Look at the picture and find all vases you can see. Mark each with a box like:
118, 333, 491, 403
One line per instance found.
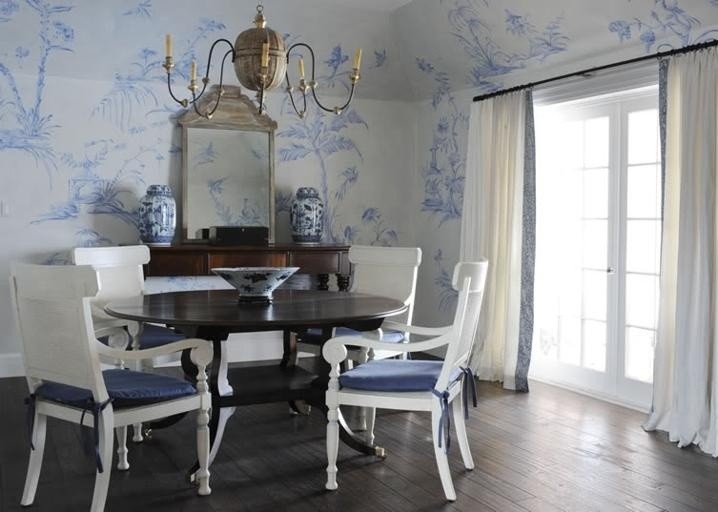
288, 186, 324, 246
136, 183, 178, 247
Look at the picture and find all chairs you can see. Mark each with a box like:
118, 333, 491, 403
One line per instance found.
295, 241, 426, 440
74, 245, 200, 442
12, 260, 214, 505
323, 256, 490, 497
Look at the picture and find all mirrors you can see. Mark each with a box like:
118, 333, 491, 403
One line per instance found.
175, 83, 278, 245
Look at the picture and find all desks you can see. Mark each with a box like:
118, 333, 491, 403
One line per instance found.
101, 286, 403, 479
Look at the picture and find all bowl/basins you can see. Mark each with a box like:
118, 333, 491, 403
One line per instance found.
211, 266, 300, 302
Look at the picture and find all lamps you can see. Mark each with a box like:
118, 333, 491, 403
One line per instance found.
161, 1, 362, 121
